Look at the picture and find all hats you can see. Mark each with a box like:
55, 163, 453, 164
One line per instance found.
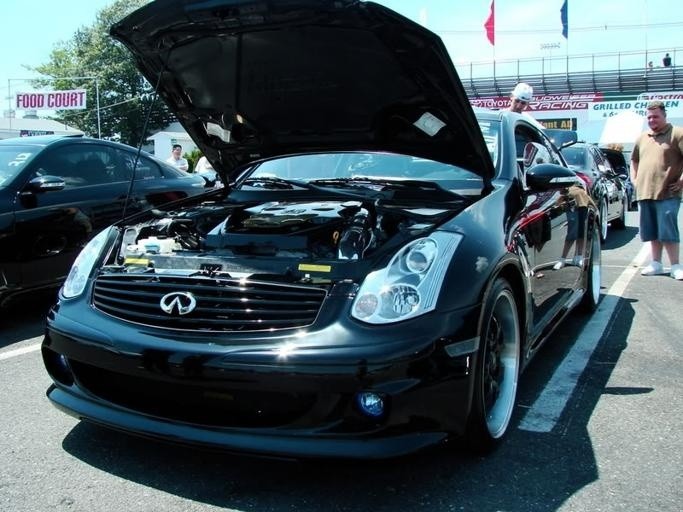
512, 82, 533, 102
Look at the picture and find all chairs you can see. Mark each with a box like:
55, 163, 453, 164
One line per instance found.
75, 158, 111, 183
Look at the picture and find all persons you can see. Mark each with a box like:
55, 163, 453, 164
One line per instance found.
662, 53, 672, 67
502, 83, 534, 113
64, 207, 93, 239
552, 186, 598, 270
630, 101, 683, 280
648, 61, 654, 71
166, 144, 218, 187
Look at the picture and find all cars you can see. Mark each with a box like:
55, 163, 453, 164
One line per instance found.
0, 134, 216, 317
600, 148, 638, 211
194, 156, 219, 184
560, 143, 629, 243
41, 0, 603, 464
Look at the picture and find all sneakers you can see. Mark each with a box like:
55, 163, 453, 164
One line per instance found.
669, 262, 683, 280
641, 259, 664, 276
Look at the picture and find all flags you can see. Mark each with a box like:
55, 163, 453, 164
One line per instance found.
484, 0, 495, 46
560, 0, 568, 40
